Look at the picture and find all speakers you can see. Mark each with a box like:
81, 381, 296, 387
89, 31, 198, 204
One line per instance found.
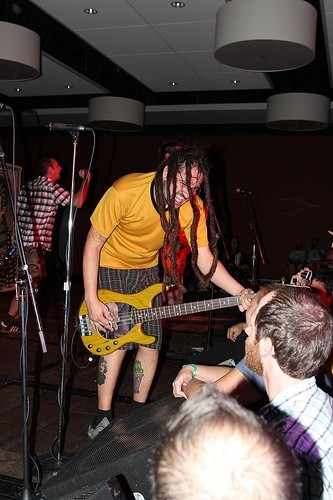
32, 391, 189, 500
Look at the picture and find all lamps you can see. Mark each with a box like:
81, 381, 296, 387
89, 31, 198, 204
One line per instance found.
265, 93, 331, 133
86, 95, 146, 136
0, 20, 43, 84
213, 0, 319, 73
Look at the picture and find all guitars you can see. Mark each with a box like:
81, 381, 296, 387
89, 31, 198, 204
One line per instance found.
76, 278, 312, 357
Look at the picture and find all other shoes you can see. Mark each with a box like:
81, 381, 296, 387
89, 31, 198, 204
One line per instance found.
4, 314, 22, 326
87, 415, 110, 438
0, 321, 21, 334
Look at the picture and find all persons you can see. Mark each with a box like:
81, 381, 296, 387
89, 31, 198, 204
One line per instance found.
307, 236, 325, 263
161, 204, 209, 305
289, 240, 307, 269
223, 238, 251, 273
80, 137, 255, 439
0, 156, 91, 332
244, 285, 333, 500
146, 381, 304, 500
172, 267, 333, 421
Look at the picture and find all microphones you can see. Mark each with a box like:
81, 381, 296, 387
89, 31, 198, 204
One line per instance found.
236, 188, 254, 195
44, 121, 92, 132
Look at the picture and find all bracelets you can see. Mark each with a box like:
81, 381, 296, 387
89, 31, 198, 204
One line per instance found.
182, 363, 199, 379
